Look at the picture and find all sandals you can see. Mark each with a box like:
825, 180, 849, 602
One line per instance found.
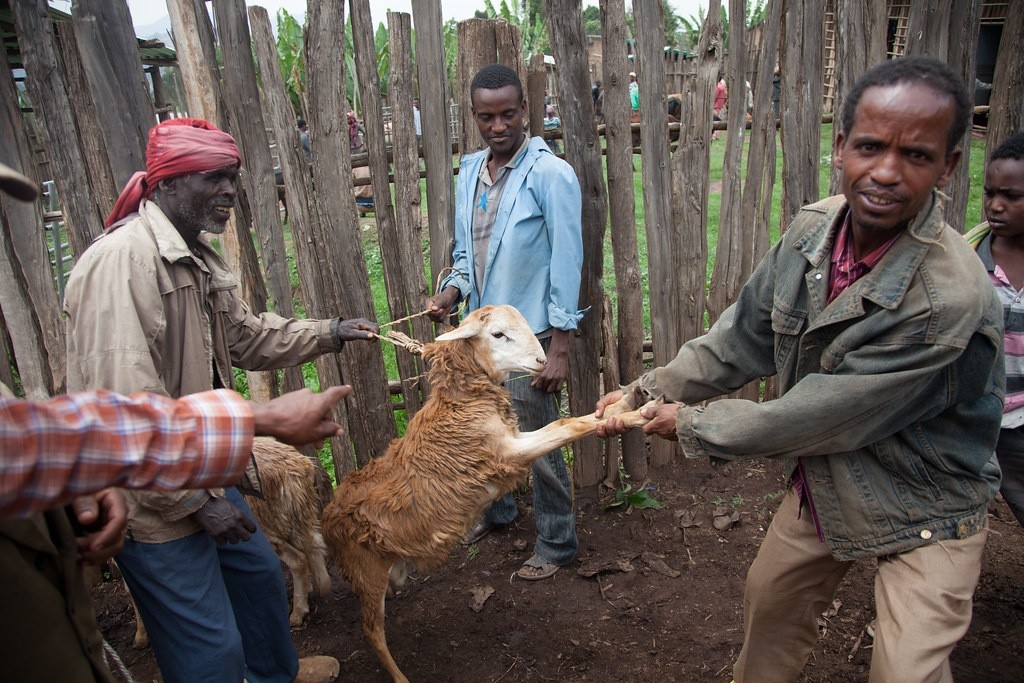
866, 611, 877, 637
518, 554, 560, 579
461, 517, 491, 545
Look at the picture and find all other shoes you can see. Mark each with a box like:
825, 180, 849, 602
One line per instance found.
292, 655, 340, 683
359, 212, 366, 217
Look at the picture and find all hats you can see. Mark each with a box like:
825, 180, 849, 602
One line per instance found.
629, 72, 636, 78
0, 161, 39, 203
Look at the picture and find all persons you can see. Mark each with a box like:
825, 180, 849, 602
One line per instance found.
0, 384, 352, 521
63, 118, 380, 683
424, 63, 593, 582
0, 162, 131, 683
962, 130, 1024, 527
288, 45, 760, 172
595, 54, 1008, 683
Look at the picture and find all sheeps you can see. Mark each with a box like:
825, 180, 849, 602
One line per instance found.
111, 435, 332, 649
321, 304, 666, 683
